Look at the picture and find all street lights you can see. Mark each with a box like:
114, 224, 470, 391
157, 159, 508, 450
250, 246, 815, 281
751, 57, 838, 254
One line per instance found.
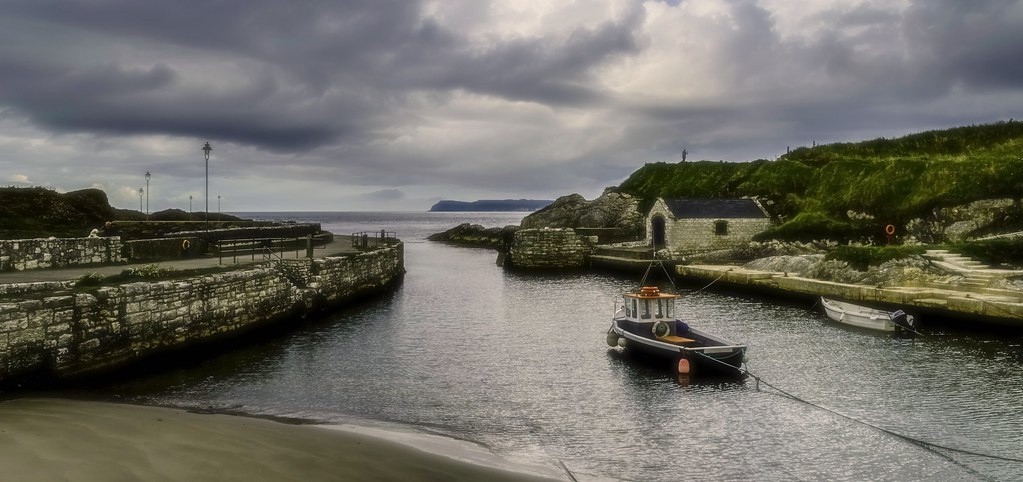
201, 141, 213, 232
190, 196, 192, 221
218, 196, 220, 221
139, 188, 144, 213
145, 172, 151, 221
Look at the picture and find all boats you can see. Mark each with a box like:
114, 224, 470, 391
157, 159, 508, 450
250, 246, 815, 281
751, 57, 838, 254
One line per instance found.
606, 286, 749, 380
820, 296, 914, 331
